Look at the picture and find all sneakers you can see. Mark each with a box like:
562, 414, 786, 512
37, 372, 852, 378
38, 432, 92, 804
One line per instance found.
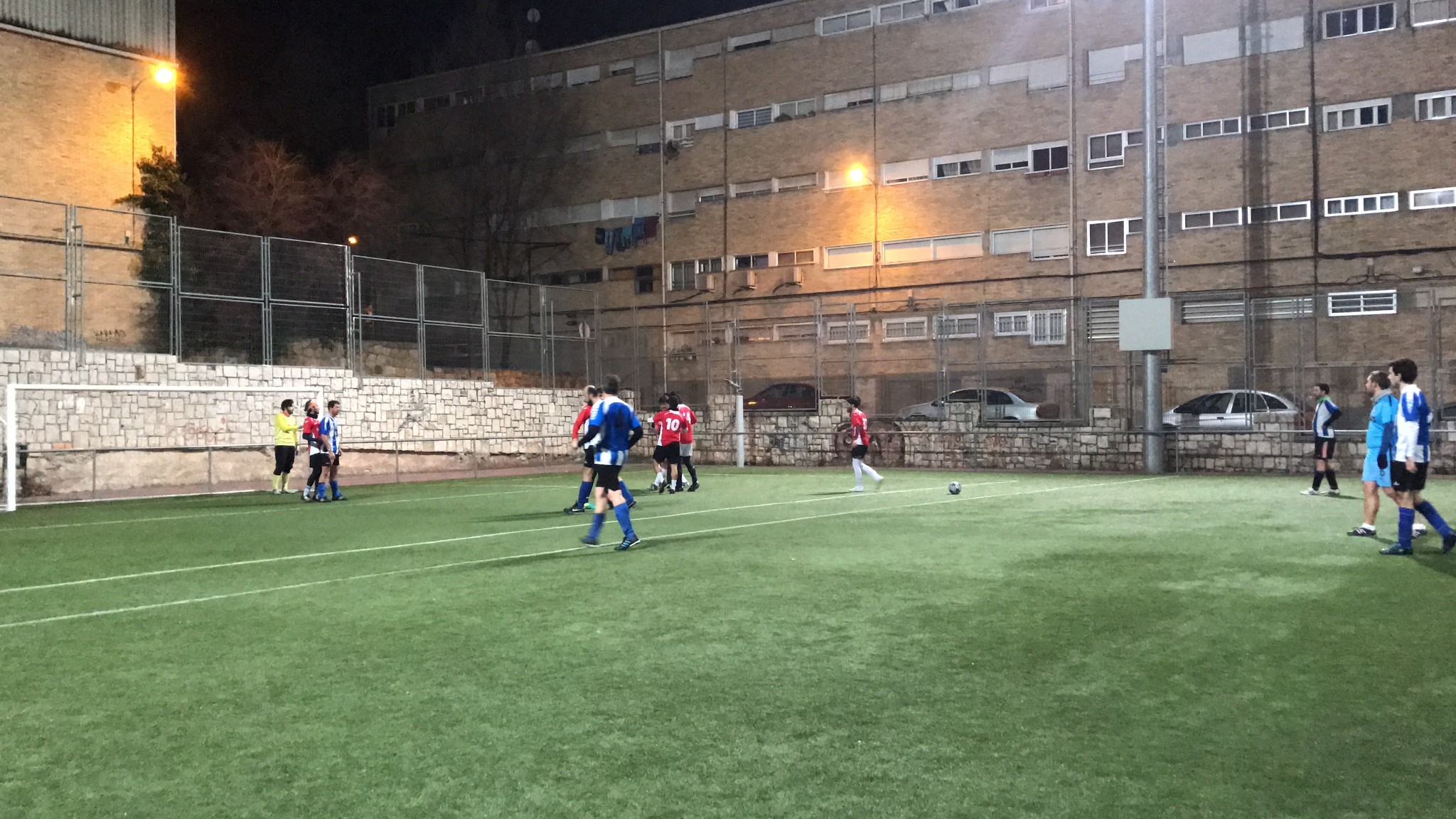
1347, 526, 1377, 537
1410, 527, 1428, 539
659, 480, 669, 493
563, 502, 585, 514
1320, 488, 1339, 497
1300, 487, 1319, 496
669, 489, 675, 494
578, 535, 599, 547
317, 496, 331, 503
847, 485, 865, 493
614, 533, 641, 551
332, 494, 348, 501
583, 502, 595, 510
674, 486, 684, 492
627, 499, 637, 510
681, 481, 690, 488
299, 492, 313, 502
1441, 529, 1456, 554
281, 488, 296, 494
1378, 542, 1414, 556
687, 480, 700, 492
271, 489, 281, 495
873, 475, 884, 492
312, 494, 328, 501
649, 483, 658, 491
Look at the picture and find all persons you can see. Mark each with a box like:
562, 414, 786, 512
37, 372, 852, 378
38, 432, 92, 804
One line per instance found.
845, 397, 883, 492
1379, 359, 1455, 555
647, 392, 700, 493
314, 400, 348, 502
563, 385, 637, 514
302, 400, 323, 501
271, 399, 302, 495
1345, 370, 1427, 540
1300, 381, 1342, 496
573, 374, 643, 551
572, 389, 614, 510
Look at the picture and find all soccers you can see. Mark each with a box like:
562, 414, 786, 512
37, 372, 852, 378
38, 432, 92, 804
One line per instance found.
948, 482, 961, 494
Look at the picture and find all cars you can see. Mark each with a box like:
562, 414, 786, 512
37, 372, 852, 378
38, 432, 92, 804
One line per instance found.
739, 379, 862, 412
1162, 388, 1306, 431
898, 385, 1057, 422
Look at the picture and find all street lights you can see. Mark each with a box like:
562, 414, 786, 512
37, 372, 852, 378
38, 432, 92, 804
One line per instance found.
846, 160, 880, 306
1416, 265, 1445, 428
127, 58, 181, 240
